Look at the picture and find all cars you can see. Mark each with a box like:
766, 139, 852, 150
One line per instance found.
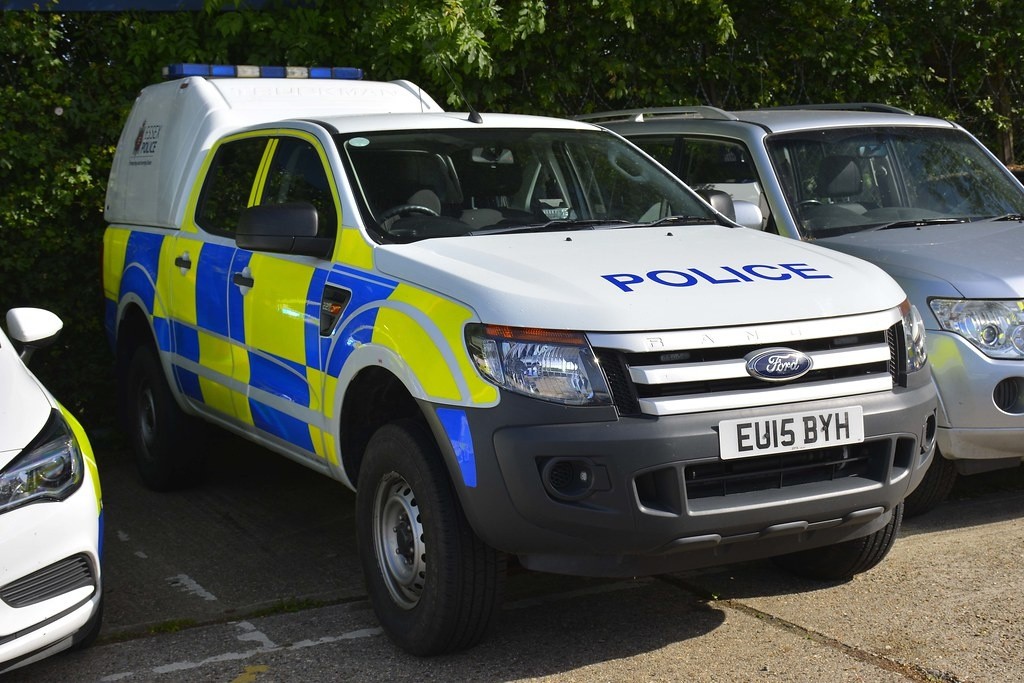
0, 305, 104, 675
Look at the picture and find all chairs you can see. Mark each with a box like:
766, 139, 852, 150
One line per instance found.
816, 155, 878, 215
458, 161, 522, 227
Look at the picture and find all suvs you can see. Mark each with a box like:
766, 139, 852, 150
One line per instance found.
507, 103, 1023, 520
103, 65, 939, 658
